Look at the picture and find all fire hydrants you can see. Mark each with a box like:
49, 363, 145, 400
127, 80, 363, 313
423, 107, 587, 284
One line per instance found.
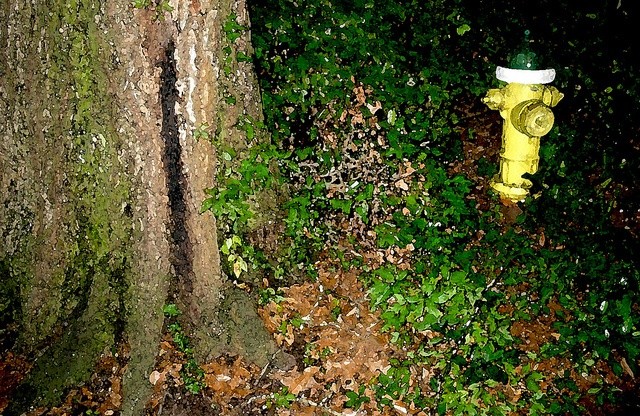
480, 52, 564, 201
